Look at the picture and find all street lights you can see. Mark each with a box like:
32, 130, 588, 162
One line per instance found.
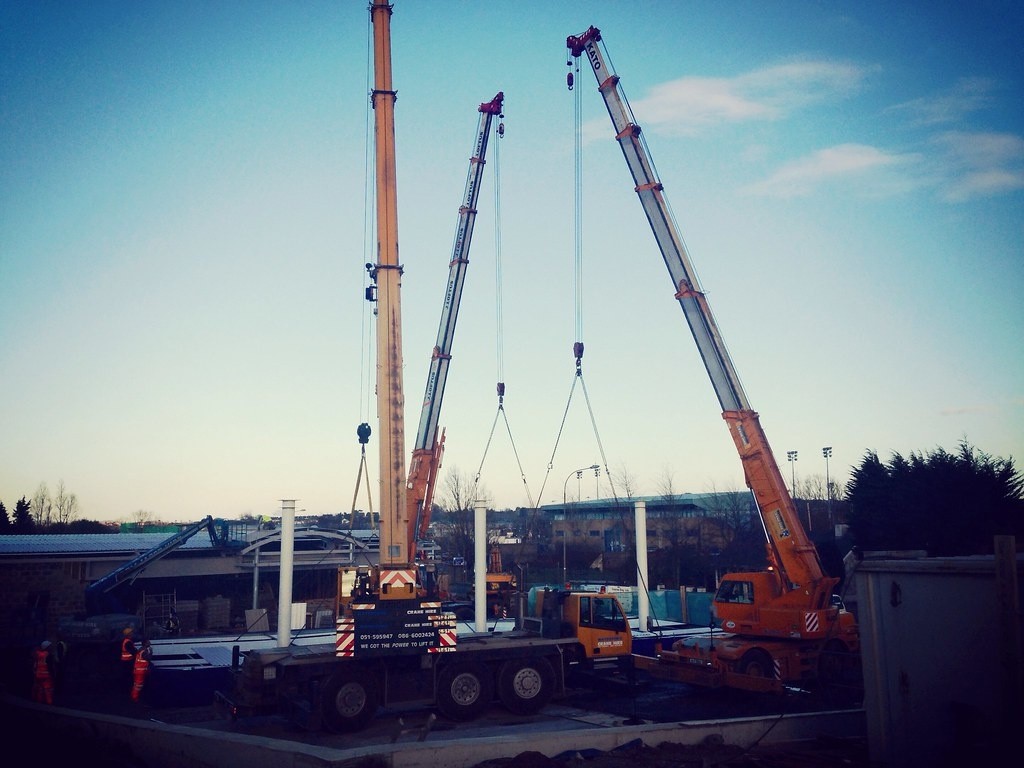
822, 444, 833, 500
786, 450, 799, 500
562, 464, 600, 583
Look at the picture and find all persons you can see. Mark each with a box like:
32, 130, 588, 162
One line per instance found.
131, 639, 153, 702
33, 641, 53, 705
120, 629, 138, 694
55, 636, 67, 676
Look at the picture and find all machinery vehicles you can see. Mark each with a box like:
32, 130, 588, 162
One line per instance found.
63, 2, 864, 724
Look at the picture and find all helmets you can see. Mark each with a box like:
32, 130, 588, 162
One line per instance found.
124, 628, 133, 636
42, 641, 52, 650
